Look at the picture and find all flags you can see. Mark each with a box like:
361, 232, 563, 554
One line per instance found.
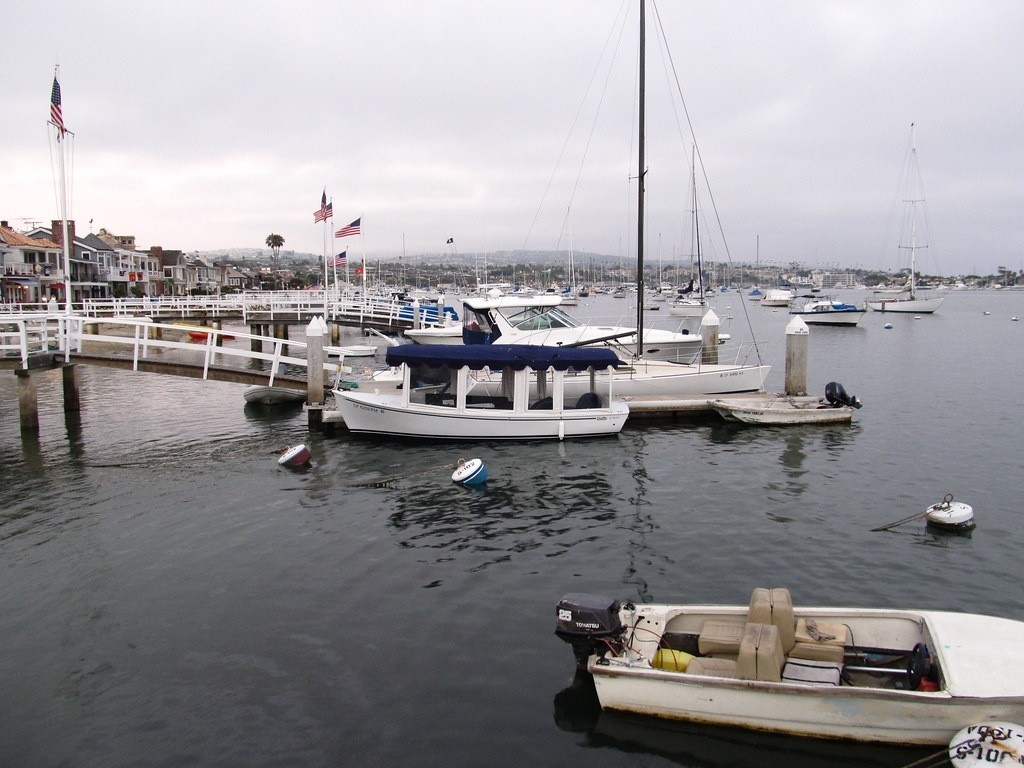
328, 251, 346, 266
50, 75, 64, 143
335, 218, 360, 237
321, 189, 327, 222
313, 203, 332, 224
355, 262, 364, 275
446, 238, 453, 243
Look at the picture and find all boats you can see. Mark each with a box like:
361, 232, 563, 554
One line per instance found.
554, 590, 1024, 750
703, 382, 863, 427
333, 341, 631, 442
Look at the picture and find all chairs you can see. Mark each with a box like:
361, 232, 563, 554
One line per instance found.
757, 625, 841, 686
686, 623, 762, 680
770, 587, 845, 662
698, 588, 771, 654
564, 393, 602, 410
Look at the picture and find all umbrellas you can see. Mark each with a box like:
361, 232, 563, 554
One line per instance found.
45, 282, 65, 289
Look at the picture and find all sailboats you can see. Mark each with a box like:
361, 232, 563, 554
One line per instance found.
286, 0, 945, 397
